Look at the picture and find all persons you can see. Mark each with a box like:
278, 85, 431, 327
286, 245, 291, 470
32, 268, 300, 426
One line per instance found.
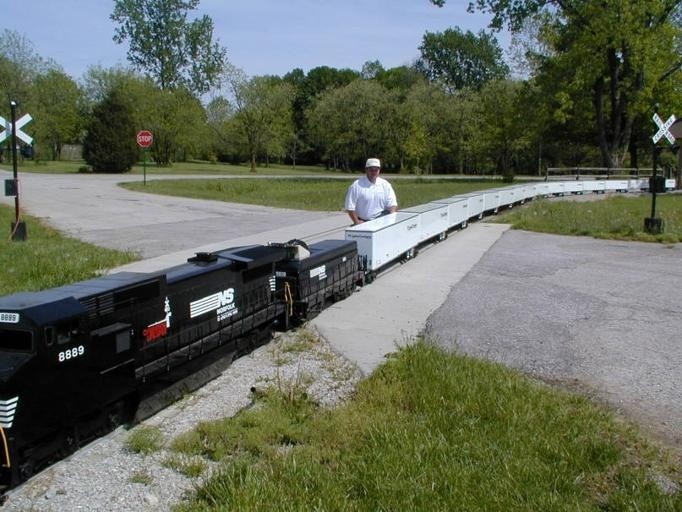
344, 158, 398, 227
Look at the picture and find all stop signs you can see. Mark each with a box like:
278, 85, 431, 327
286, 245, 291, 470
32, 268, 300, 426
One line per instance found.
137, 131, 153, 147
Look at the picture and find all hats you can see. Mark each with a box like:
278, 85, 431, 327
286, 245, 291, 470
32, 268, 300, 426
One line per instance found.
366, 158, 381, 168
